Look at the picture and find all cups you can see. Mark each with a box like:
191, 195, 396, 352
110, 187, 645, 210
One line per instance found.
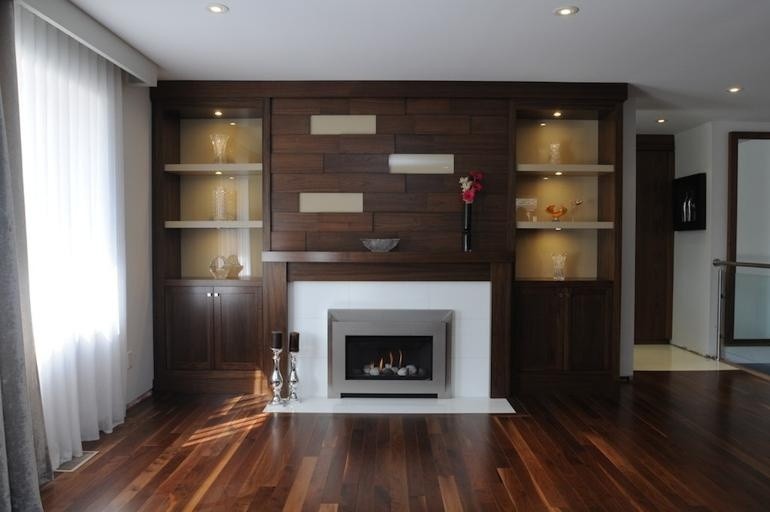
208, 133, 230, 165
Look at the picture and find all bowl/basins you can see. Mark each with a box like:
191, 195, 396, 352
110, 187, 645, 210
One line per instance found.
360, 238, 401, 252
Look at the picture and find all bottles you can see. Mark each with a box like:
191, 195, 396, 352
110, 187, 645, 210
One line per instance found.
212, 171, 227, 221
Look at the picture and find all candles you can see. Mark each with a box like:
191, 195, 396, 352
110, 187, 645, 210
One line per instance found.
270, 328, 301, 351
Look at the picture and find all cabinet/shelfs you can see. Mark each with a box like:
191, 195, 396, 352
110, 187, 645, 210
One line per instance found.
509, 84, 629, 390
670, 173, 707, 232
150, 81, 273, 396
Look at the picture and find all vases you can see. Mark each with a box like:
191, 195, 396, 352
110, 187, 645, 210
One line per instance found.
463, 201, 472, 251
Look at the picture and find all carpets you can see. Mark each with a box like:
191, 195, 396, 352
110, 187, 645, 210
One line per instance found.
634, 343, 740, 372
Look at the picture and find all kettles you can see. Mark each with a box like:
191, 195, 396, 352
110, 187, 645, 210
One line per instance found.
682, 192, 697, 224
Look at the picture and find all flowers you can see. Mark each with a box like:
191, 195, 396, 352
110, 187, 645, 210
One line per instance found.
458, 170, 485, 203
